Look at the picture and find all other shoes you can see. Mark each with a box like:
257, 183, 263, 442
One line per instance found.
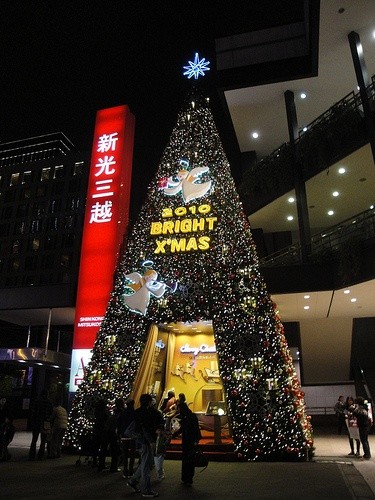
355, 452, 360, 455
126, 482, 138, 492
348, 452, 354, 455
123, 473, 133, 478
160, 473, 167, 480
363, 455, 371, 458
142, 491, 159, 497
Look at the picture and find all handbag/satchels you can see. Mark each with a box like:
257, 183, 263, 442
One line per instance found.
195, 445, 208, 467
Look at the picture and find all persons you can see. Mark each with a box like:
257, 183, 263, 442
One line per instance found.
334, 395, 371, 459
92, 392, 202, 498
26, 389, 68, 459
